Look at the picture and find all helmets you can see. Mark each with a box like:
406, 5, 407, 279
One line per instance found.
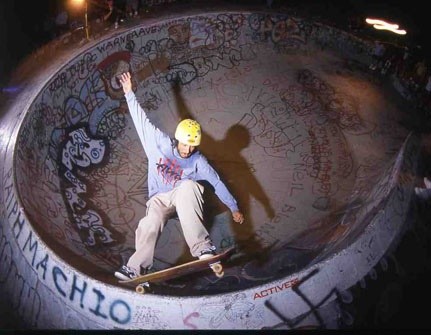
175, 119, 203, 146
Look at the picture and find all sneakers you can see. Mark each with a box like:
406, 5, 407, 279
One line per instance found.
114, 265, 149, 288
200, 248, 217, 259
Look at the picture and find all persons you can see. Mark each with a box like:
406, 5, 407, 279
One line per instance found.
113, 71, 245, 280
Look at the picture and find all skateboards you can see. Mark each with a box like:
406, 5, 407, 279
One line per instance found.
116, 245, 237, 294
